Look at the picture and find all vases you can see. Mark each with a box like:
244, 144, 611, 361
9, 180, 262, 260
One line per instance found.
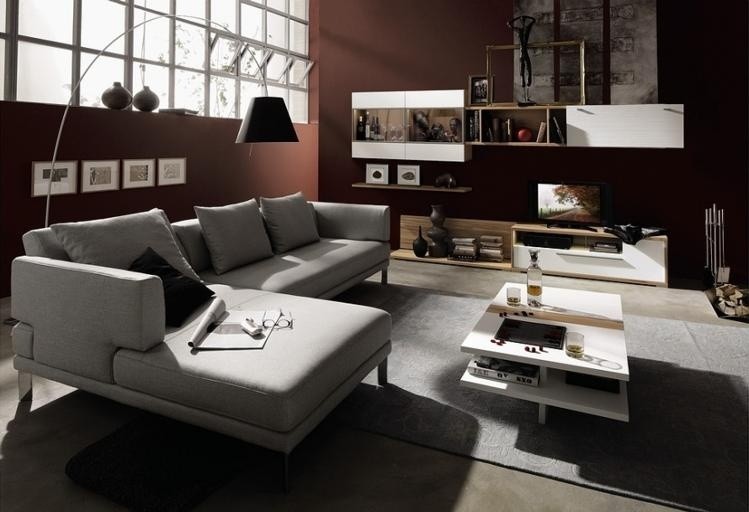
103, 82, 132, 111
133, 86, 159, 113
413, 204, 448, 257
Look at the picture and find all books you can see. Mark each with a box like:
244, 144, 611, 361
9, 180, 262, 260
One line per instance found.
565, 371, 620, 395
465, 109, 565, 145
452, 234, 504, 263
589, 240, 619, 253
467, 354, 542, 387
188, 295, 282, 354
494, 317, 566, 349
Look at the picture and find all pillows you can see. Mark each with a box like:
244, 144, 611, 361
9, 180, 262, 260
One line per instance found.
260, 190, 322, 255
194, 198, 275, 275
51, 206, 205, 285
128, 245, 215, 328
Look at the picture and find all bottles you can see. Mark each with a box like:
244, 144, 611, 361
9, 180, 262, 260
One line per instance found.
526, 247, 544, 306
357, 110, 380, 140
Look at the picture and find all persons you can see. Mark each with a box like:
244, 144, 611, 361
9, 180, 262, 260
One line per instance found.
507, 15, 536, 87
448, 118, 460, 142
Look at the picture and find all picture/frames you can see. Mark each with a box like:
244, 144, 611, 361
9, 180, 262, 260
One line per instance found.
157, 157, 186, 186
81, 160, 120, 194
366, 164, 389, 184
32, 160, 78, 198
122, 159, 156, 189
397, 165, 421, 186
468, 74, 493, 106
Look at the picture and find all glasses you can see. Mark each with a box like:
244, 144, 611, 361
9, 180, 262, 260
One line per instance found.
261, 311, 293, 328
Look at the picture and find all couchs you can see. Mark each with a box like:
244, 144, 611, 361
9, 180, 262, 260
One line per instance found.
10, 201, 392, 494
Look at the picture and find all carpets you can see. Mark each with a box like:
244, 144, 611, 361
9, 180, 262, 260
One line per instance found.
326, 279, 749, 512
66, 411, 258, 512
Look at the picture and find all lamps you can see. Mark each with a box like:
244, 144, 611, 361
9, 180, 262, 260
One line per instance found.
44, 13, 299, 227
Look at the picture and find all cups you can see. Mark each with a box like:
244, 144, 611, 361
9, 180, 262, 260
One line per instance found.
507, 287, 521, 307
564, 331, 586, 360
381, 122, 404, 142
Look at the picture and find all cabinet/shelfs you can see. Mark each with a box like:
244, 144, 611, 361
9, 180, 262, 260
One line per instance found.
464, 101, 685, 150
351, 89, 472, 162
510, 224, 668, 288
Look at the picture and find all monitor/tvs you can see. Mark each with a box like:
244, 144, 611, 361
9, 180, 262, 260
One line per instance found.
528, 179, 613, 232
